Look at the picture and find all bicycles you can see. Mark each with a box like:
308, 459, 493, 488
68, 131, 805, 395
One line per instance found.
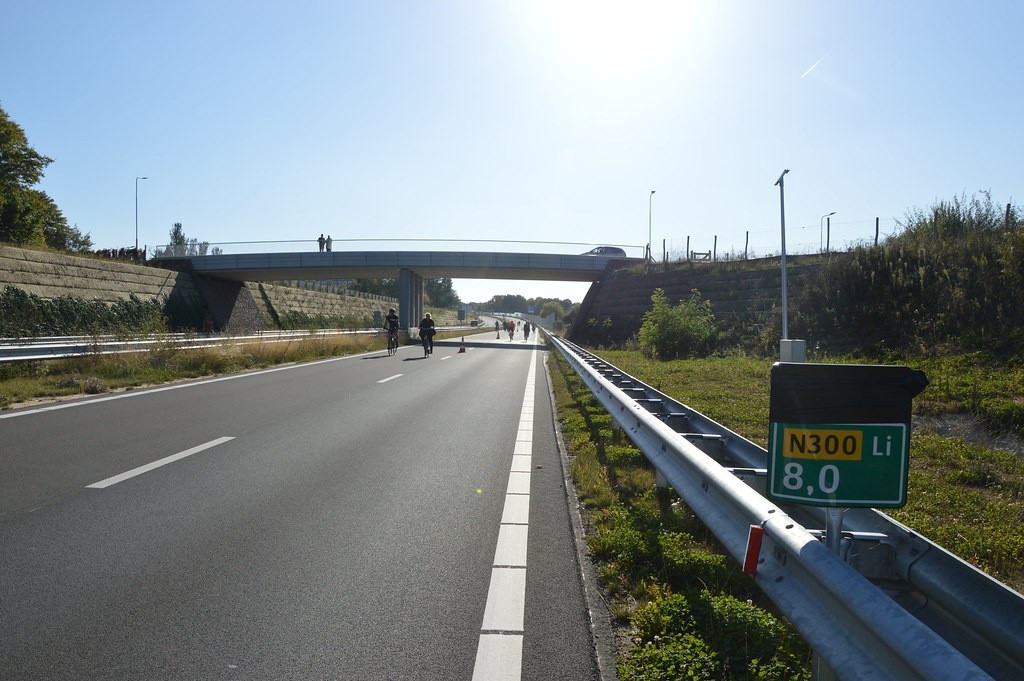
383, 326, 398, 356
418, 327, 432, 358
495, 326, 535, 342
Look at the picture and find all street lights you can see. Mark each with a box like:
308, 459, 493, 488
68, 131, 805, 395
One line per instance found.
135, 176, 149, 249
648, 191, 657, 249
774, 168, 792, 339
820, 212, 836, 253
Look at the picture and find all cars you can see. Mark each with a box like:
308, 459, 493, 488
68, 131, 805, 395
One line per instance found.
581, 246, 627, 259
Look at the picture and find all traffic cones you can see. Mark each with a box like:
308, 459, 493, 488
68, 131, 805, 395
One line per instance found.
457, 335, 467, 353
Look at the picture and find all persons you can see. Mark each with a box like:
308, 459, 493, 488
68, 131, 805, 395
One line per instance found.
494, 319, 536, 340
317, 234, 326, 252
418, 313, 435, 354
383, 308, 400, 350
325, 235, 331, 252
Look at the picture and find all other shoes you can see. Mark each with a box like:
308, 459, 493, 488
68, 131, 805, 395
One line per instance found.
396, 343, 399, 347
